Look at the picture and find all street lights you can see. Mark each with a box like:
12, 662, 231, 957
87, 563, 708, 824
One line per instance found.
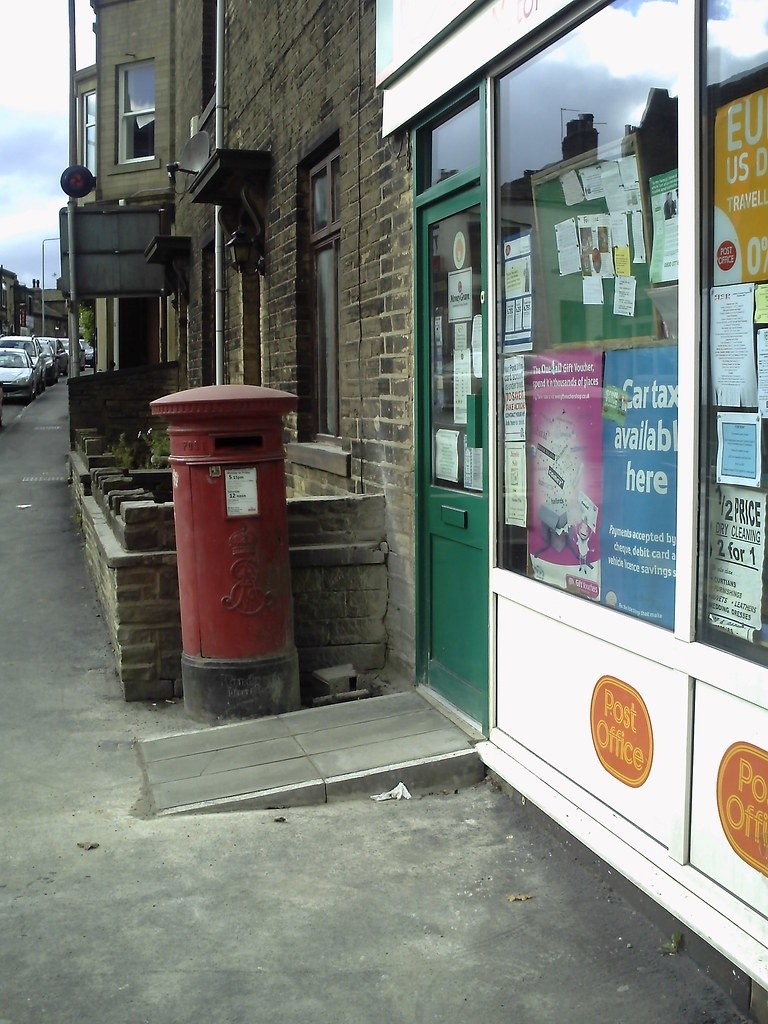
41, 237, 61, 335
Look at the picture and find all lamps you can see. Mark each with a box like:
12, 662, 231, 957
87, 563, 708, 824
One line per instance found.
225, 225, 267, 276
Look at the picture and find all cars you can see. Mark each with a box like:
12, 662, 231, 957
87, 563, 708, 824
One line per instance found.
0, 348, 37, 406
37, 337, 94, 385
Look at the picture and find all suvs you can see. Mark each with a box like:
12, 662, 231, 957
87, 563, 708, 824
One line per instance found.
0, 335, 47, 390
